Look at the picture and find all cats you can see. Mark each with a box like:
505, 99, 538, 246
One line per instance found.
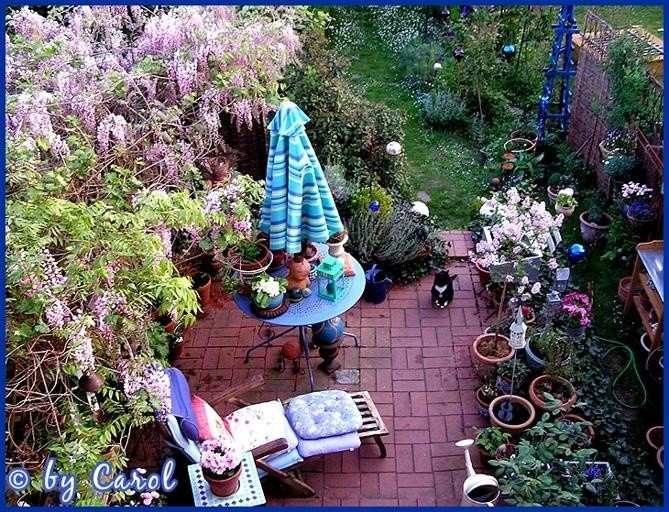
431, 270, 458, 309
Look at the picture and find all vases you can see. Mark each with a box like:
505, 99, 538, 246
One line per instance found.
505, 130, 539, 156
617, 277, 631, 302
152, 229, 349, 359
472, 332, 595, 465
202, 462, 243, 497
646, 426, 664, 470
208, 156, 230, 181
548, 137, 657, 246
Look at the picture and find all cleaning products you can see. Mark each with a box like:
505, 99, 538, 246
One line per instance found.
509, 304, 527, 349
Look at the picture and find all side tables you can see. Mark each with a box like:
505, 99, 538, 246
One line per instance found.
187, 451, 267, 507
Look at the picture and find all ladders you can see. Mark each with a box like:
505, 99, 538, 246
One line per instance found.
536, 3, 581, 143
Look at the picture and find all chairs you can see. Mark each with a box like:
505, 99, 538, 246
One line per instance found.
155, 366, 391, 495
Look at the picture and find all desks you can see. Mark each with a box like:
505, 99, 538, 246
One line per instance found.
230, 249, 366, 393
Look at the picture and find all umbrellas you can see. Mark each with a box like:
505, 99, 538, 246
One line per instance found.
259, 100, 345, 351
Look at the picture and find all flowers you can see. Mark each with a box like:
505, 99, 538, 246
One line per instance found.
197, 438, 243, 478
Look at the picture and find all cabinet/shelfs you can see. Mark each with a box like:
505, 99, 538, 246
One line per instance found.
624, 240, 664, 352
